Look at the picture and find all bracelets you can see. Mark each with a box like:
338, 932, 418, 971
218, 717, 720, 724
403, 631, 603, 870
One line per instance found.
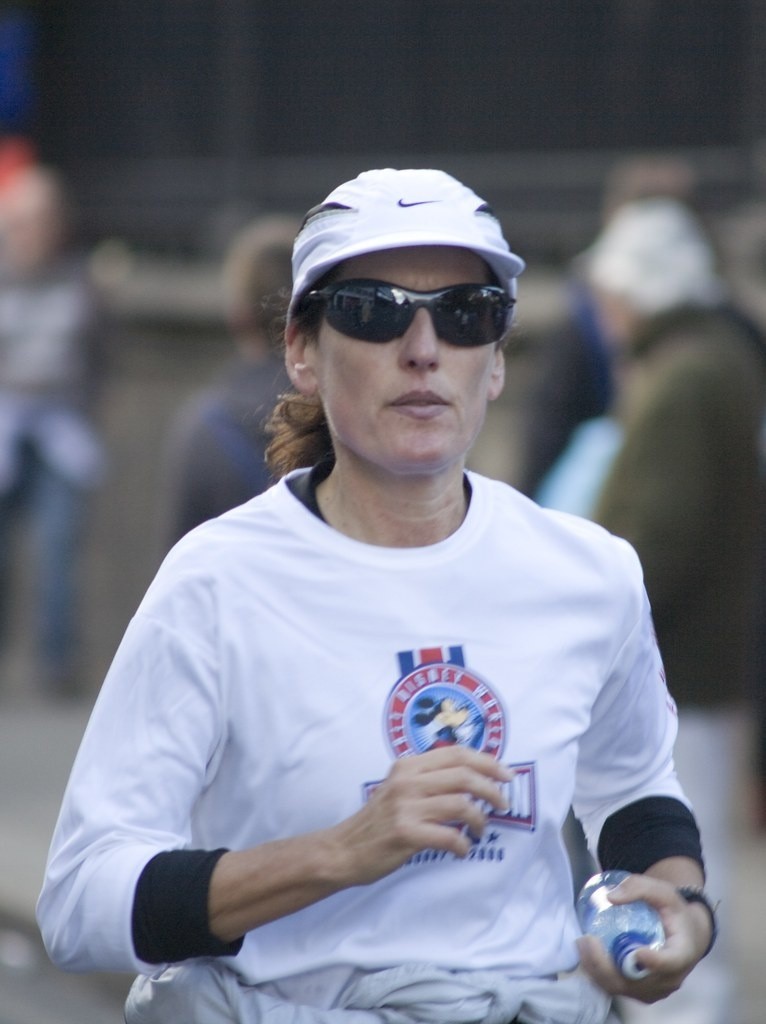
679, 886, 718, 958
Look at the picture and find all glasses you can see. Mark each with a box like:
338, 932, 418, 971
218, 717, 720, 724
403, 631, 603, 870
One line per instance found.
304, 277, 517, 347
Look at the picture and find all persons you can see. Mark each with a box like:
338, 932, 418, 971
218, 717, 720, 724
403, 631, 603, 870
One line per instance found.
0, 163, 100, 705
39, 167, 711, 1024
159, 211, 326, 566
513, 149, 766, 1024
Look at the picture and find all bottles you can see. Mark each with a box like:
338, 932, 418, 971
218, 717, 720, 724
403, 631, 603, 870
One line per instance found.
576, 871, 664, 979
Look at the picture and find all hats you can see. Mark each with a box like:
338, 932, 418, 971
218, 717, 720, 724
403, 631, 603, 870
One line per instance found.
282, 168, 524, 349
577, 196, 712, 309
